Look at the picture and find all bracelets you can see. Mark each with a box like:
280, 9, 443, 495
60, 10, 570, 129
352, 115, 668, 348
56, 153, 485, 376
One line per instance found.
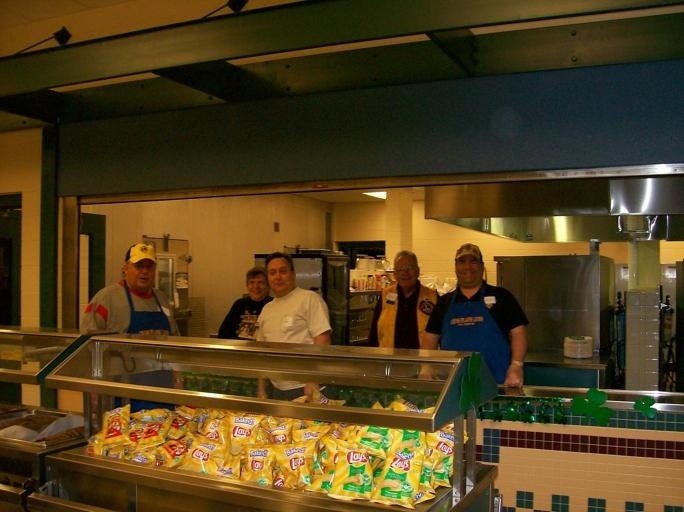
510, 359, 525, 368
173, 377, 185, 384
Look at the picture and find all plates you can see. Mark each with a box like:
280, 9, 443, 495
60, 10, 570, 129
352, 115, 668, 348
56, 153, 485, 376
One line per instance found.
562, 336, 594, 359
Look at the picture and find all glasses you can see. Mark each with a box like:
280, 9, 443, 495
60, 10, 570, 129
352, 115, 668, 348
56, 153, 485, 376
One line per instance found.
127, 262, 157, 272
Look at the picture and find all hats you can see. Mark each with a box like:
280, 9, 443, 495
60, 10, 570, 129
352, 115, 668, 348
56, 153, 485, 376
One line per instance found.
125, 243, 158, 266
454, 243, 483, 263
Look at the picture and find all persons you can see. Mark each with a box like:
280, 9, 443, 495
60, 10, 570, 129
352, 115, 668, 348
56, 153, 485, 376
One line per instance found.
255, 249, 334, 402
217, 266, 275, 341
369, 250, 441, 352
81, 243, 185, 414
418, 243, 531, 390
